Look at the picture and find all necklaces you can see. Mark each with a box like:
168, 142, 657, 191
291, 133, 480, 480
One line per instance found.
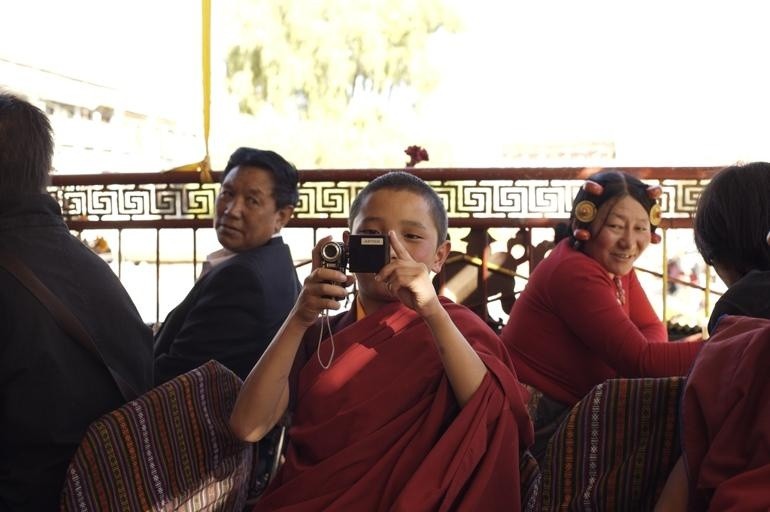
614, 276, 626, 306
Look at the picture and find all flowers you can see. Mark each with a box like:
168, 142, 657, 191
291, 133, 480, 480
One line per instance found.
405, 145, 429, 167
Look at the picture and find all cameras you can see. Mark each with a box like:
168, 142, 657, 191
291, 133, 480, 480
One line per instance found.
319, 234, 390, 301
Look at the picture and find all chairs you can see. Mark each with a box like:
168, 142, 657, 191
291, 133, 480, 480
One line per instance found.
521, 383, 543, 422
62, 360, 253, 511
536, 376, 690, 511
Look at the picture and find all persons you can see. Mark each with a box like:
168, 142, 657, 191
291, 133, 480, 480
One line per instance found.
0, 93, 155, 512
153, 148, 308, 480
651, 162, 770, 512
498, 170, 712, 459
228, 170, 535, 512
